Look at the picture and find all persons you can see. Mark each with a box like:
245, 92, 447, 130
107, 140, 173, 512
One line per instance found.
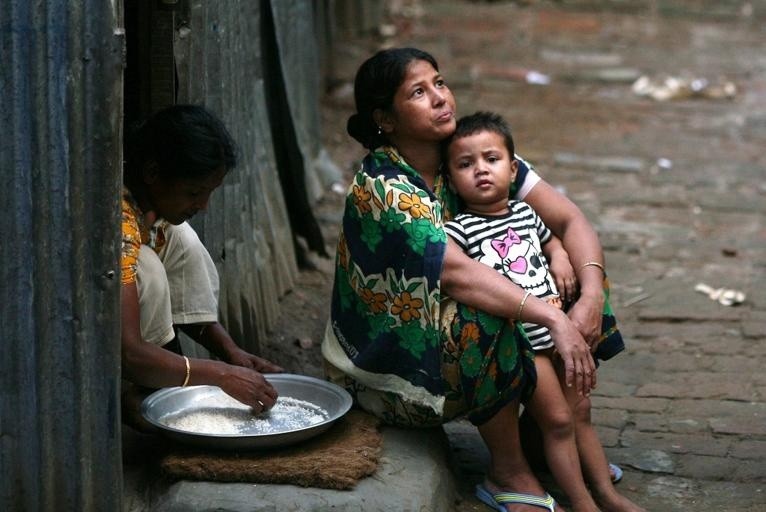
444, 112, 648, 512
321, 46, 625, 511
119, 102, 286, 429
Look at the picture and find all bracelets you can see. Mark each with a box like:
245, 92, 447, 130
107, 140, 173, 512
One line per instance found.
518, 292, 531, 321
197, 323, 208, 344
576, 261, 608, 282
180, 355, 190, 389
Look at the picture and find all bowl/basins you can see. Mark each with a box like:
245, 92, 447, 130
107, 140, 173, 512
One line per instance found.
139, 375, 352, 453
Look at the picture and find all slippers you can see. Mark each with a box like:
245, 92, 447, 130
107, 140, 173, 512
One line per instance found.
606, 463, 623, 482
475, 482, 558, 512
